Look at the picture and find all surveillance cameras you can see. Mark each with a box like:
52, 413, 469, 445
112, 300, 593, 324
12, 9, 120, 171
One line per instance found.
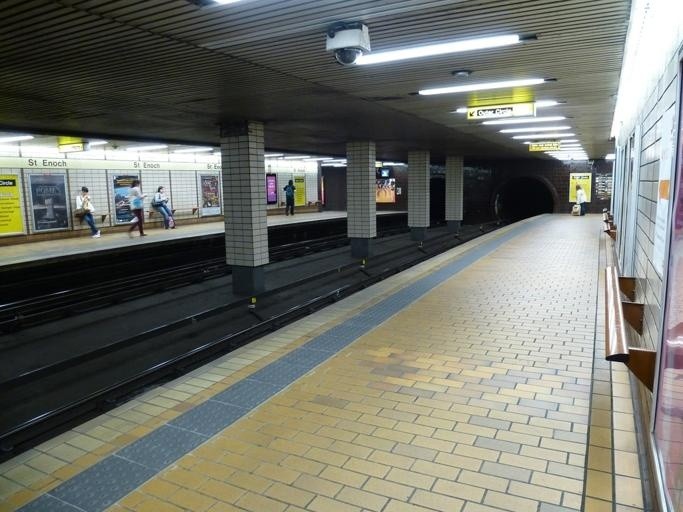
326, 23, 371, 66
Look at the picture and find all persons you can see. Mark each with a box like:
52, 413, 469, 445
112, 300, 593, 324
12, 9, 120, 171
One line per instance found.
575, 181, 586, 215
150, 186, 175, 229
126, 179, 148, 239
283, 179, 296, 216
75, 186, 101, 238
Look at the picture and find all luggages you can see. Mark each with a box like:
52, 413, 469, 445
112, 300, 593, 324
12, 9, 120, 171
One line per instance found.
572, 204, 581, 216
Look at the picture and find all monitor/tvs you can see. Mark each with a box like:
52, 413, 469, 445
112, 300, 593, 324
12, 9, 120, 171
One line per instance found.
380, 168, 391, 179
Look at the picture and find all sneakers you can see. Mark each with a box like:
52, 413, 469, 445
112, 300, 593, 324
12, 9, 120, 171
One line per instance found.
140, 233, 147, 236
93, 230, 100, 238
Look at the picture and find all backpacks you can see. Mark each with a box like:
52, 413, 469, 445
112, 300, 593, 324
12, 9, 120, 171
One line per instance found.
286, 186, 292, 195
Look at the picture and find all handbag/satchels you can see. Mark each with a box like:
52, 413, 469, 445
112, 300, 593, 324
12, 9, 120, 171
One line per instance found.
151, 199, 163, 207
133, 198, 141, 207
168, 217, 174, 228
74, 208, 85, 218
